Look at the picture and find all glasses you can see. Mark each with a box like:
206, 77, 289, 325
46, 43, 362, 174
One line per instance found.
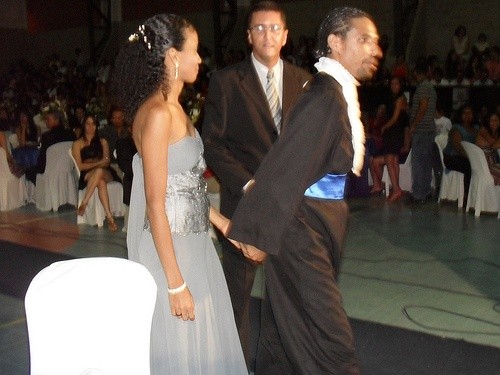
250, 24, 285, 33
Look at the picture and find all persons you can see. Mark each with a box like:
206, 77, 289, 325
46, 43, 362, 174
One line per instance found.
280, 35, 314, 75
110, 14, 248, 375
72, 115, 117, 231
25, 109, 78, 186
345, 25, 500, 207
200, 0, 314, 326
0, 47, 112, 130
102, 111, 137, 231
13, 110, 42, 167
226, 7, 383, 375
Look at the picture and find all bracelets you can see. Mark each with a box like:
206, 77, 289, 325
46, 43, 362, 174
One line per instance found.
168, 282, 187, 294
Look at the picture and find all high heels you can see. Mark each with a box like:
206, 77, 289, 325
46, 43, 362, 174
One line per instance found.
386, 190, 402, 204
108, 220, 117, 232
368, 187, 382, 196
76, 202, 86, 216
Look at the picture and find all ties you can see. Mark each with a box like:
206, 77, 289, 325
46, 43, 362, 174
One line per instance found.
265, 69, 282, 136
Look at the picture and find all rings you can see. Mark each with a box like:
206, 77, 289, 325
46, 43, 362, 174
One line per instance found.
176, 313, 182, 316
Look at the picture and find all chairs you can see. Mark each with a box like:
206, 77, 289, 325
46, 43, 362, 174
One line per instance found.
367, 125, 500, 217
0, 132, 125, 228
24, 256, 157, 375
